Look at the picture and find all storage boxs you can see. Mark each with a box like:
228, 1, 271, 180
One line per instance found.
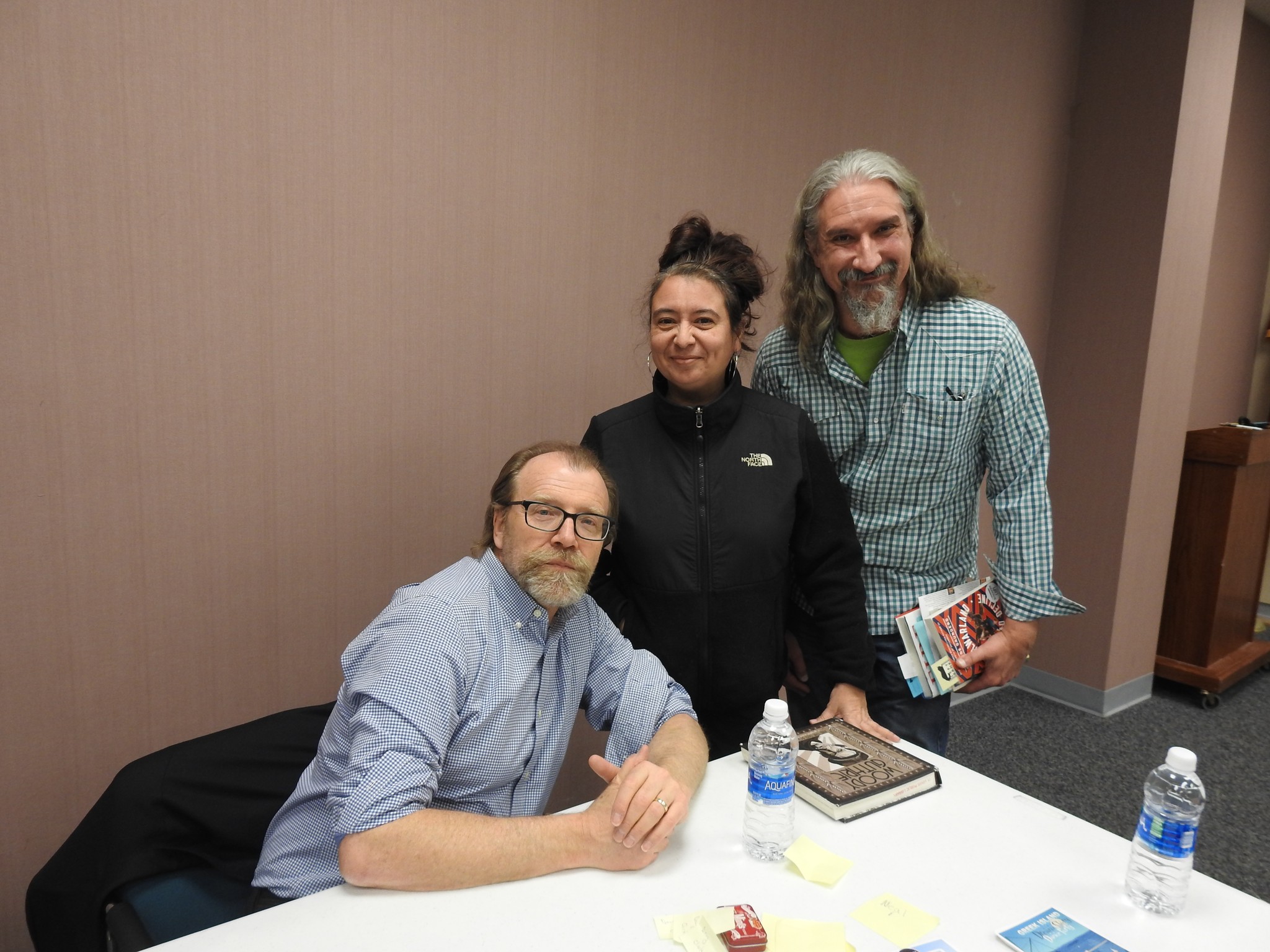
716, 904, 768, 952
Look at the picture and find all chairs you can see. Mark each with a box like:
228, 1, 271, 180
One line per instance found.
26, 700, 340, 952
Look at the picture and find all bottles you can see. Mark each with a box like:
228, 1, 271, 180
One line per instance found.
740, 698, 799, 866
1124, 745, 1207, 916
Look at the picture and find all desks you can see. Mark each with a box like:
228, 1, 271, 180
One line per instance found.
141, 737, 1270, 952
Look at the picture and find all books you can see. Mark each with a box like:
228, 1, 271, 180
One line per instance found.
895, 576, 1004, 700
739, 716, 943, 825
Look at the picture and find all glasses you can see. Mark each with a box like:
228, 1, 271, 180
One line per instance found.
500, 500, 615, 541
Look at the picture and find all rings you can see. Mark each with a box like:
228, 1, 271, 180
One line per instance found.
1026, 655, 1031, 664
654, 798, 668, 812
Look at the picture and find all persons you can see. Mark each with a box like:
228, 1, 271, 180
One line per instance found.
251, 441, 709, 914
751, 149, 1086, 756
580, 209, 900, 762
